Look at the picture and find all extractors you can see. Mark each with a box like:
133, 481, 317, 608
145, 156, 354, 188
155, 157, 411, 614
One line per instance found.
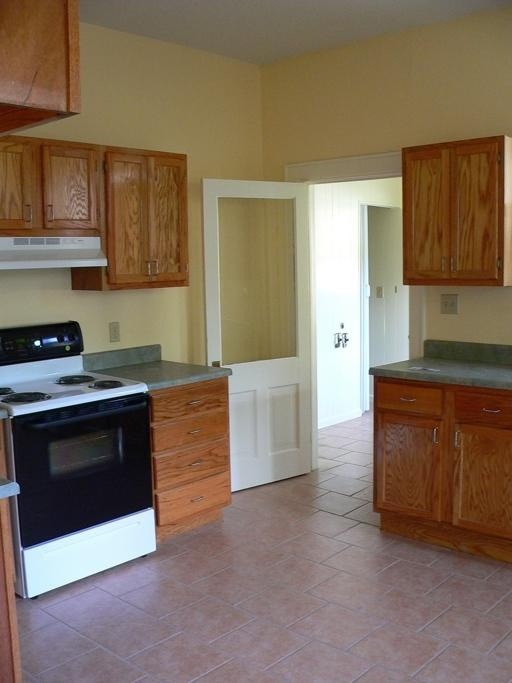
0, 236, 110, 272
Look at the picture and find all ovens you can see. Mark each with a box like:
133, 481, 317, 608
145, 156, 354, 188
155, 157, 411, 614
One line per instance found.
6, 393, 156, 600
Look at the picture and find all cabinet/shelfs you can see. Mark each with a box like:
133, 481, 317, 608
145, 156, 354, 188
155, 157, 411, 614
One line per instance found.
149, 377, 231, 544
373, 377, 512, 563
71, 151, 189, 289
402, 142, 511, 287
0, 142, 98, 238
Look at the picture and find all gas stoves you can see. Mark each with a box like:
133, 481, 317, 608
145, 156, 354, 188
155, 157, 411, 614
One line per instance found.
0, 372, 149, 416
